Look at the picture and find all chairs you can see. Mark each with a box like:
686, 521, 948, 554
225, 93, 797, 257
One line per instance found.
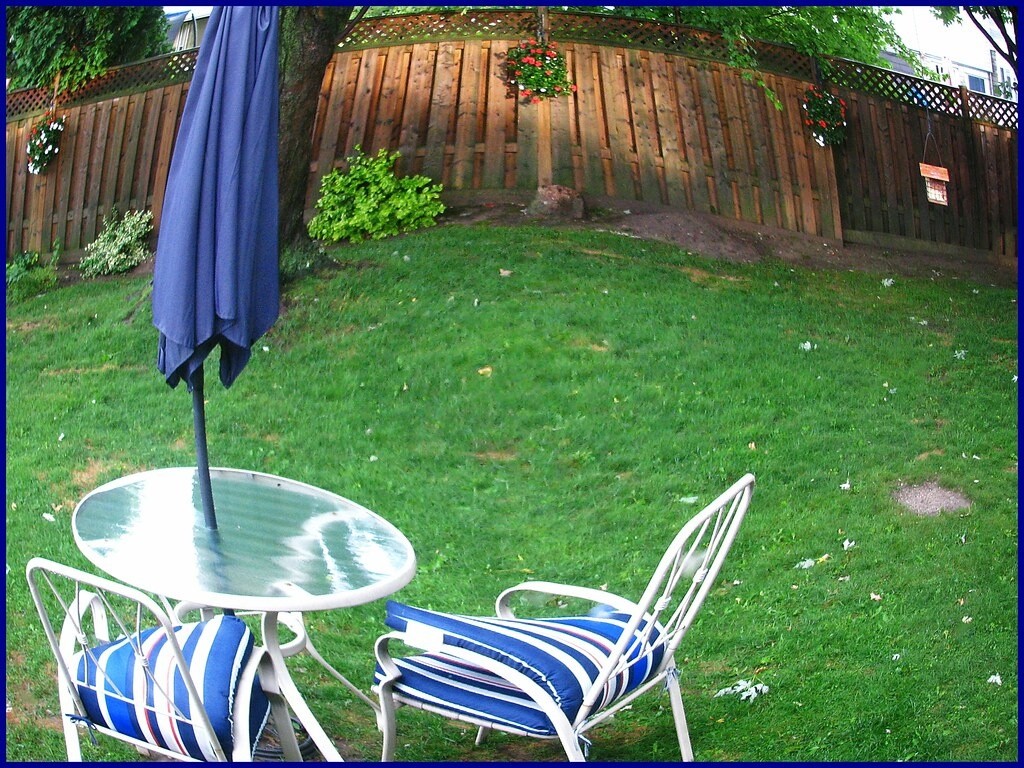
371, 471, 756, 764
25, 558, 303, 764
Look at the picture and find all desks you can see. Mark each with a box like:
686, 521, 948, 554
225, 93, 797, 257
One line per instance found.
75, 465, 416, 764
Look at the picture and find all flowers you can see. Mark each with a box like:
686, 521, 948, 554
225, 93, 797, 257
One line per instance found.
803, 84, 849, 148
504, 35, 578, 105
25, 109, 68, 175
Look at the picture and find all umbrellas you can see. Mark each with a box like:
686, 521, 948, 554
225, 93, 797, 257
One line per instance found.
149, 4, 284, 614
